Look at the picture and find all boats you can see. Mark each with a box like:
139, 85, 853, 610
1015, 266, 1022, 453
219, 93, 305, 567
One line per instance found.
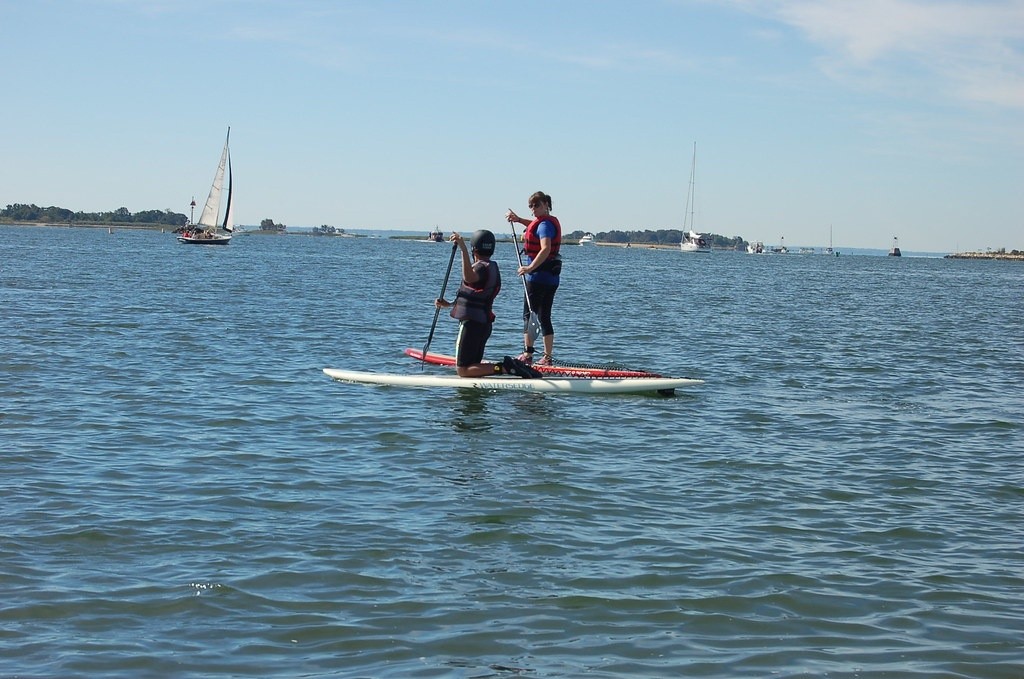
428, 231, 444, 242
824, 247, 833, 255
745, 241, 766, 254
579, 232, 596, 246
888, 236, 901, 257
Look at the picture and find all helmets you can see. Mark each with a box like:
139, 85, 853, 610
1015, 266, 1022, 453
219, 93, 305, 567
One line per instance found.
470, 230, 495, 255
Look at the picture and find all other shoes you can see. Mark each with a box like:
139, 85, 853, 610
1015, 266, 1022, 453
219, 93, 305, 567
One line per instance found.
514, 355, 532, 366
501, 356, 542, 379
535, 357, 552, 365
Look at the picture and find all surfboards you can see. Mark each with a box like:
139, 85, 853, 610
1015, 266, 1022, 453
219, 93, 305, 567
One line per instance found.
404, 347, 663, 379
321, 367, 707, 393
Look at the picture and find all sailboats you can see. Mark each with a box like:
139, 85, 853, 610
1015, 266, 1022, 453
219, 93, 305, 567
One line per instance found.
175, 126, 234, 245
681, 141, 713, 253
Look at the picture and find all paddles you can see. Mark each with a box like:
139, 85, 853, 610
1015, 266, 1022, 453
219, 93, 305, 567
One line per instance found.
421, 239, 459, 372
509, 218, 542, 342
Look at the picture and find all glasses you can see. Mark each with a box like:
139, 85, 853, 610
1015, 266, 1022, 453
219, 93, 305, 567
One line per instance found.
529, 203, 541, 208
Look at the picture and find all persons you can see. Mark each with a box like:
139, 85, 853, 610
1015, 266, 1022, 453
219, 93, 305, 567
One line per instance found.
505, 191, 562, 366
434, 229, 543, 379
